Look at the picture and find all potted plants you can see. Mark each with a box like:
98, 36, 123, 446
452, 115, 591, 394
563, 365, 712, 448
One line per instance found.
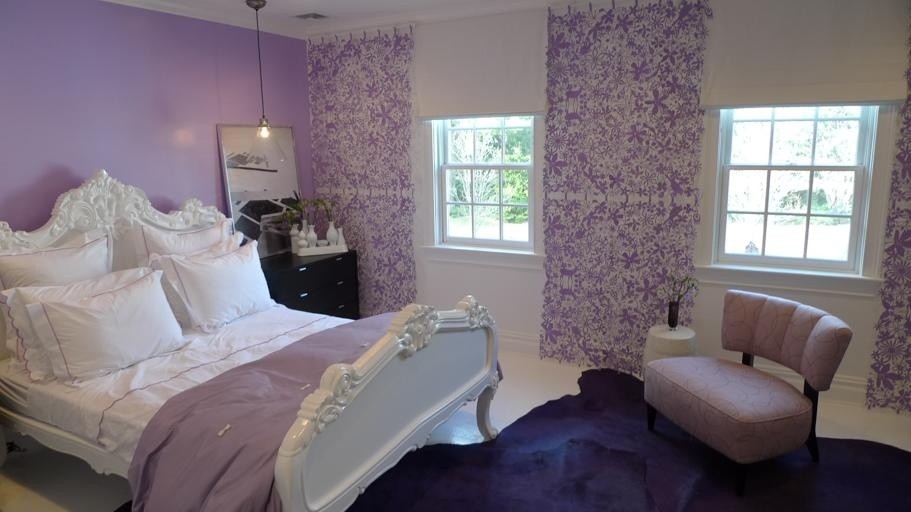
283, 198, 338, 254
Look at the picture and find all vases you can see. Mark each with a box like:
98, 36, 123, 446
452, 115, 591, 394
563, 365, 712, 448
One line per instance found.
668, 300, 680, 332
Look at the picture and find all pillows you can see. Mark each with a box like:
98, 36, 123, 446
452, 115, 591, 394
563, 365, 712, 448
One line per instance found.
131, 217, 236, 265
1, 269, 186, 383
1, 227, 114, 286
158, 240, 277, 334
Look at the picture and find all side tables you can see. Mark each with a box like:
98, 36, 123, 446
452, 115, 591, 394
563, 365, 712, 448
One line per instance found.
644, 324, 697, 376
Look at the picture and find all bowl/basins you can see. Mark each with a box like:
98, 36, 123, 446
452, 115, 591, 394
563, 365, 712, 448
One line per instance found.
317, 239, 329, 247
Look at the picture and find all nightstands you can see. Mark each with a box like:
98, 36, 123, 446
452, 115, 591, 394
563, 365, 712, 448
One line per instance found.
255, 248, 361, 319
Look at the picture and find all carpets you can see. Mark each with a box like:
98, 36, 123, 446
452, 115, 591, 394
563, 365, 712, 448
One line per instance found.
346, 366, 911, 511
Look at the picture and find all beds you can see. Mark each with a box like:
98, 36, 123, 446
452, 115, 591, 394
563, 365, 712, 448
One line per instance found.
0, 167, 506, 512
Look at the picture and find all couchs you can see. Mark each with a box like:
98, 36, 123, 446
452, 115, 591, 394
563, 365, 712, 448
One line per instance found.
643, 286, 856, 492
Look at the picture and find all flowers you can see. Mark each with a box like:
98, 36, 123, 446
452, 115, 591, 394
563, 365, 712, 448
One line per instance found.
656, 275, 700, 307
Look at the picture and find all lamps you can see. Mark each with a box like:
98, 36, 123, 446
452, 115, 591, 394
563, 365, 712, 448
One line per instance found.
246, 1, 287, 166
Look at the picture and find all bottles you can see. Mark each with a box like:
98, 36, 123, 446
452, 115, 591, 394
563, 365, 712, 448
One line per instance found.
337, 227, 346, 247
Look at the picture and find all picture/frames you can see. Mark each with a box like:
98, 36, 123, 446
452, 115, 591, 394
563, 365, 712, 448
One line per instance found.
215, 121, 303, 260
